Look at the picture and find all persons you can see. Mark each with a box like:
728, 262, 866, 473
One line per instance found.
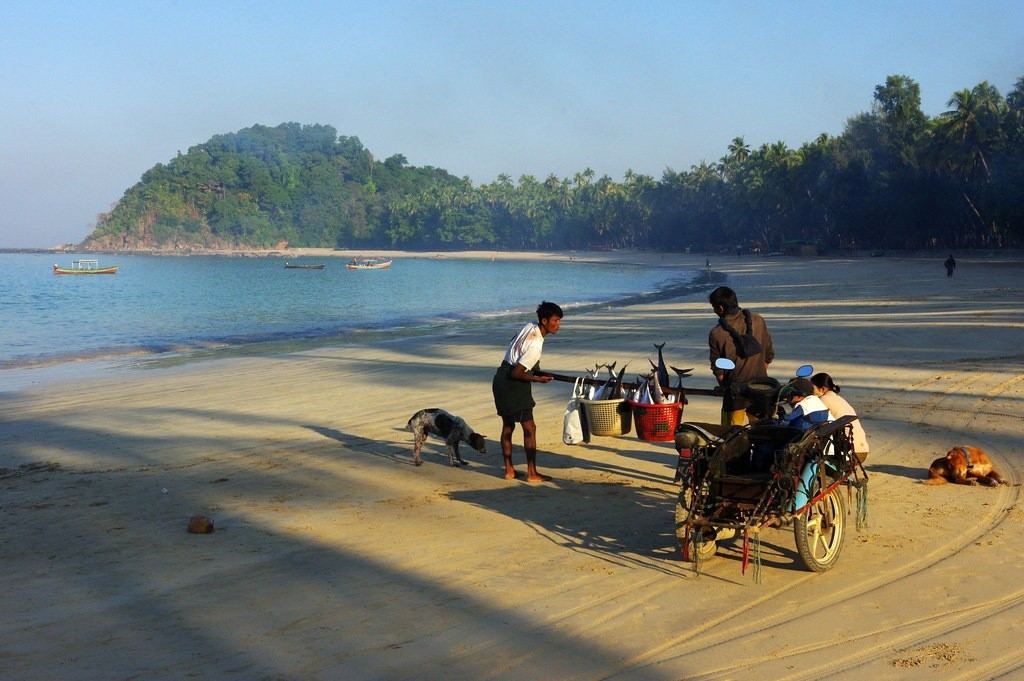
353, 257, 357, 266
945, 255, 955, 278
811, 372, 869, 465
787, 377, 851, 471
492, 301, 563, 482
708, 287, 776, 426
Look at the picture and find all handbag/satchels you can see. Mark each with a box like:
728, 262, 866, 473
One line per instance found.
562, 377, 590, 444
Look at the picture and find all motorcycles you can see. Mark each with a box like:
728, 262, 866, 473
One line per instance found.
673, 357, 814, 516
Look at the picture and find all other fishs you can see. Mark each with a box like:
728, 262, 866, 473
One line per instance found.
587, 361, 630, 400
630, 340, 691, 406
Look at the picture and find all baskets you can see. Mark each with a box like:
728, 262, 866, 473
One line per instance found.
580, 395, 632, 436
629, 397, 685, 442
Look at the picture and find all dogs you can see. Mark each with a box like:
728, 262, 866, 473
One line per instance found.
917, 444, 1011, 488
405, 408, 488, 469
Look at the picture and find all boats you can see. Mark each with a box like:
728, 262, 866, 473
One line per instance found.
53, 260, 119, 274
346, 258, 392, 269
284, 262, 325, 270
